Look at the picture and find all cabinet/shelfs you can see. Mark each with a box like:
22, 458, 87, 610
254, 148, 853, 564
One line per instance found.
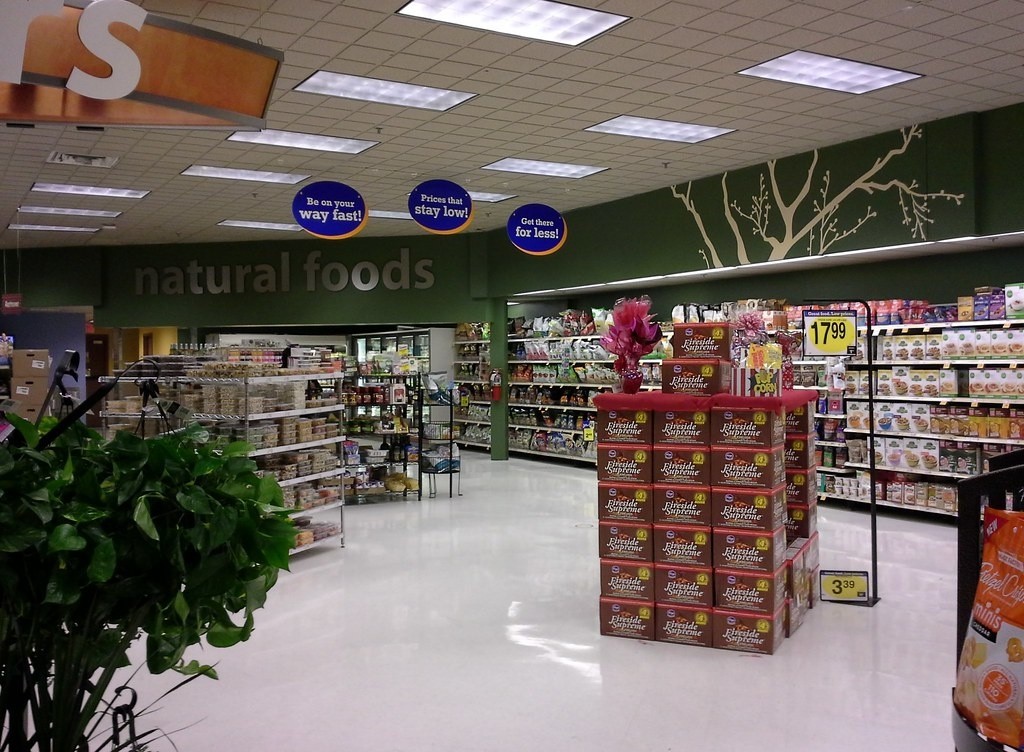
451, 318, 1024, 525
421, 386, 463, 500
97, 374, 343, 554
0, 333, 17, 452
339, 371, 424, 503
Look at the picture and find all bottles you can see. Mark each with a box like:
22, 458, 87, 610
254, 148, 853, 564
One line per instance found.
228, 346, 285, 362
383, 406, 394, 430
170, 344, 217, 355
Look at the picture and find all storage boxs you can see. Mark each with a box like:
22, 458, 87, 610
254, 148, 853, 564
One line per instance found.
12, 349, 50, 377
594, 324, 822, 654
12, 401, 49, 425
11, 377, 48, 401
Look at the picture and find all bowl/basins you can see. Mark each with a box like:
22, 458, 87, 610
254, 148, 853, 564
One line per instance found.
907, 455, 919, 466
922, 456, 937, 469
879, 381, 1024, 397
875, 453, 883, 464
883, 343, 1023, 359
916, 424, 927, 431
879, 422, 891, 430
897, 423, 909, 430
888, 455, 901, 465
